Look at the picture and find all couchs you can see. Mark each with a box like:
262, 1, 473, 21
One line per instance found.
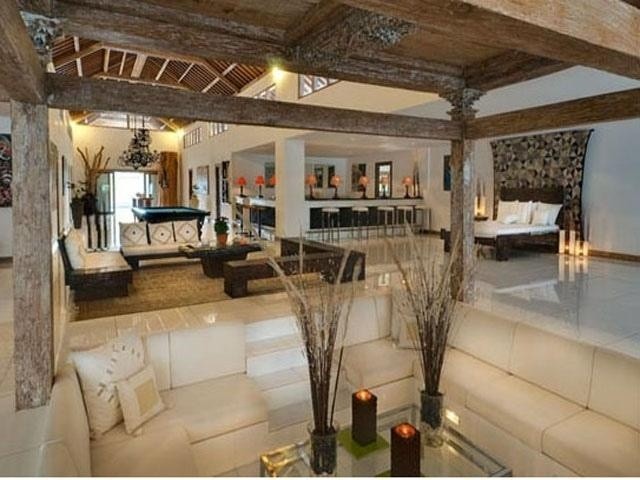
37, 299, 272, 476
334, 294, 639, 475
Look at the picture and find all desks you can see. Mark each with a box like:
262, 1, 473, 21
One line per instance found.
130, 205, 210, 243
262, 403, 513, 476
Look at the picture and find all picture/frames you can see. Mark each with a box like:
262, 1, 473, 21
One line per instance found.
130, 205, 210, 243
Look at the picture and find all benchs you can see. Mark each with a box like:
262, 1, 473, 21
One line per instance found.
119, 219, 213, 271
223, 235, 366, 294
59, 223, 133, 303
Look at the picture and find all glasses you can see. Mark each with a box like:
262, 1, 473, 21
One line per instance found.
236, 174, 275, 198
118, 110, 164, 171
306, 172, 412, 198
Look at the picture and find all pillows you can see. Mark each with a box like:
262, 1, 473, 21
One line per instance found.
497, 196, 566, 227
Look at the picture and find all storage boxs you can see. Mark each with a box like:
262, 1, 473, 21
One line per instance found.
223, 235, 366, 294
59, 223, 133, 303
119, 219, 213, 271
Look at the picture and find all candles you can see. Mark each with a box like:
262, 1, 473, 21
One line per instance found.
555, 228, 590, 260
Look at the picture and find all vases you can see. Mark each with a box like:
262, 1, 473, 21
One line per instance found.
306, 419, 341, 476
418, 387, 446, 448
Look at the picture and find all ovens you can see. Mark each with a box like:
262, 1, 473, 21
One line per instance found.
439, 178, 569, 259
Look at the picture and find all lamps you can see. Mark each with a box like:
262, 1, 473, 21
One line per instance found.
118, 110, 164, 171
236, 174, 275, 198
306, 172, 412, 198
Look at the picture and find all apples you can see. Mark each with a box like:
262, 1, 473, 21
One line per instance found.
555, 228, 590, 260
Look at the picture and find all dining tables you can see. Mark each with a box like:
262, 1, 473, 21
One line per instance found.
215, 217, 228, 245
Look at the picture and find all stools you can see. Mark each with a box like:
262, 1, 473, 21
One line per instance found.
320, 205, 433, 244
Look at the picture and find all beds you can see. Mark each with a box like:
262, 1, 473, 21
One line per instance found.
439, 178, 569, 259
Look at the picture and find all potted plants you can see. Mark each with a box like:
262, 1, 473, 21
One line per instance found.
215, 217, 228, 245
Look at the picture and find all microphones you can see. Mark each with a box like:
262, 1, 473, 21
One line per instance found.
320, 205, 433, 244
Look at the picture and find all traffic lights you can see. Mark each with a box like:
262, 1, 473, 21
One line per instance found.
497, 196, 566, 227
